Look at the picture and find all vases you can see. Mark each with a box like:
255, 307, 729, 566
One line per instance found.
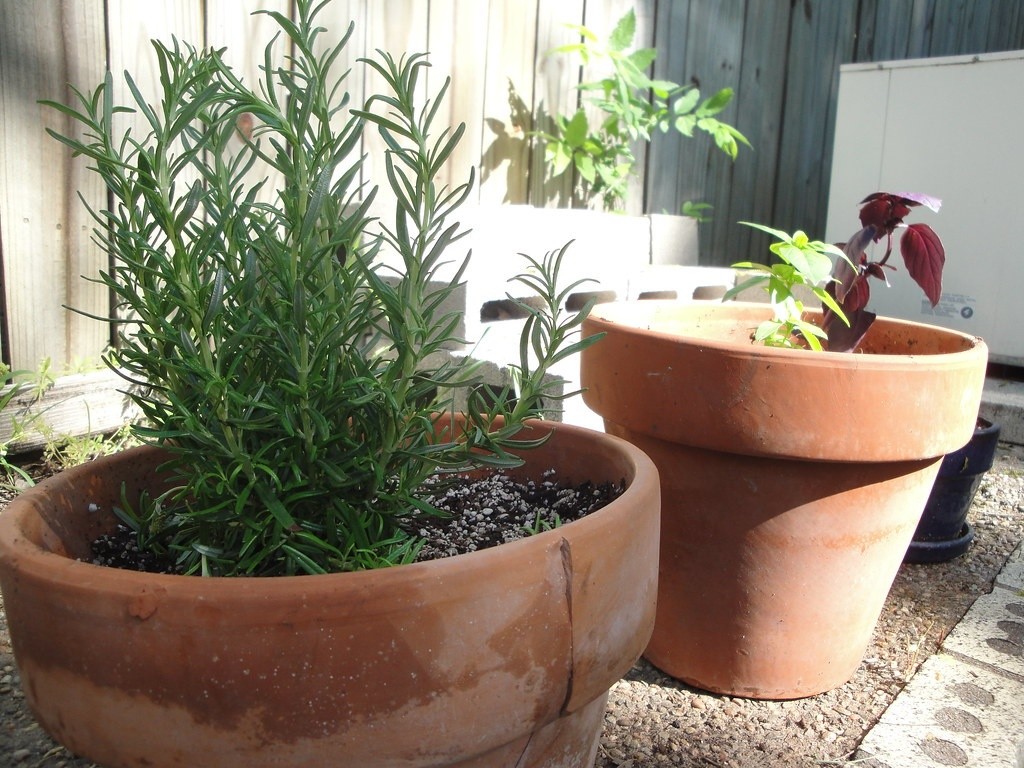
911, 415, 1001, 542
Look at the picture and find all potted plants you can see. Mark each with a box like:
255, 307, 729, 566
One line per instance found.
578, 192, 990, 702
0, 0, 667, 768
